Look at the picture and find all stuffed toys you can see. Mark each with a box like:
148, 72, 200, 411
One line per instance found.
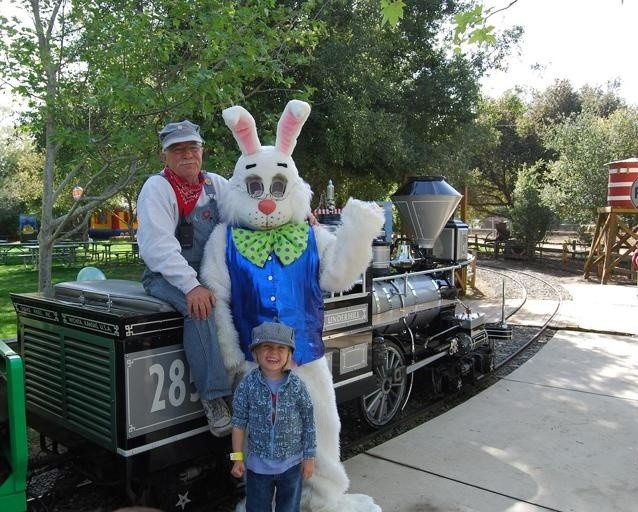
197, 98, 386, 512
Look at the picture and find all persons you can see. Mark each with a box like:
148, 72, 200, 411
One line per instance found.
135, 120, 323, 438
229, 320, 317, 512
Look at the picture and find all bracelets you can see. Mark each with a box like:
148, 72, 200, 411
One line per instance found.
228, 452, 244, 462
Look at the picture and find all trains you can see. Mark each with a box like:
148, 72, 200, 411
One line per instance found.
1, 174, 515, 511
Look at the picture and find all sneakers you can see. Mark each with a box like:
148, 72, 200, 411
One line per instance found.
201, 399, 233, 437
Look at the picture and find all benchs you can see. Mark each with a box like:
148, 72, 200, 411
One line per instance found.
0, 236, 144, 274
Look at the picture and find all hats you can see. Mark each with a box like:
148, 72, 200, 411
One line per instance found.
159, 119, 205, 152
248, 321, 296, 355
